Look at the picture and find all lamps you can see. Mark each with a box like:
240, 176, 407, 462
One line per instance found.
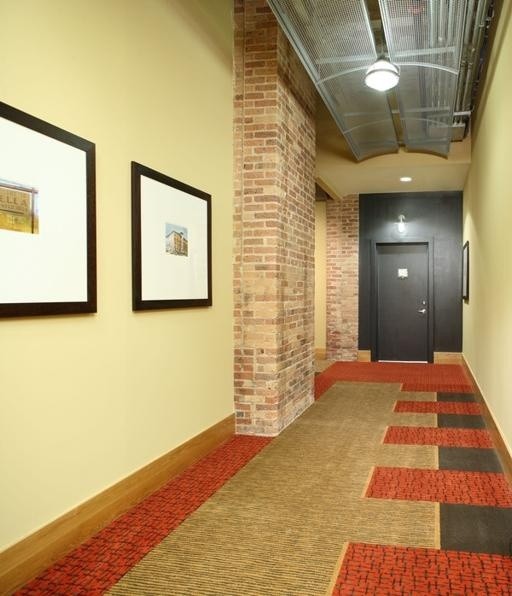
366, 40, 401, 92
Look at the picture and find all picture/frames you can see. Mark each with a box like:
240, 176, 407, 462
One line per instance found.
460, 240, 470, 298
130, 160, 213, 311
0, 99, 97, 316
